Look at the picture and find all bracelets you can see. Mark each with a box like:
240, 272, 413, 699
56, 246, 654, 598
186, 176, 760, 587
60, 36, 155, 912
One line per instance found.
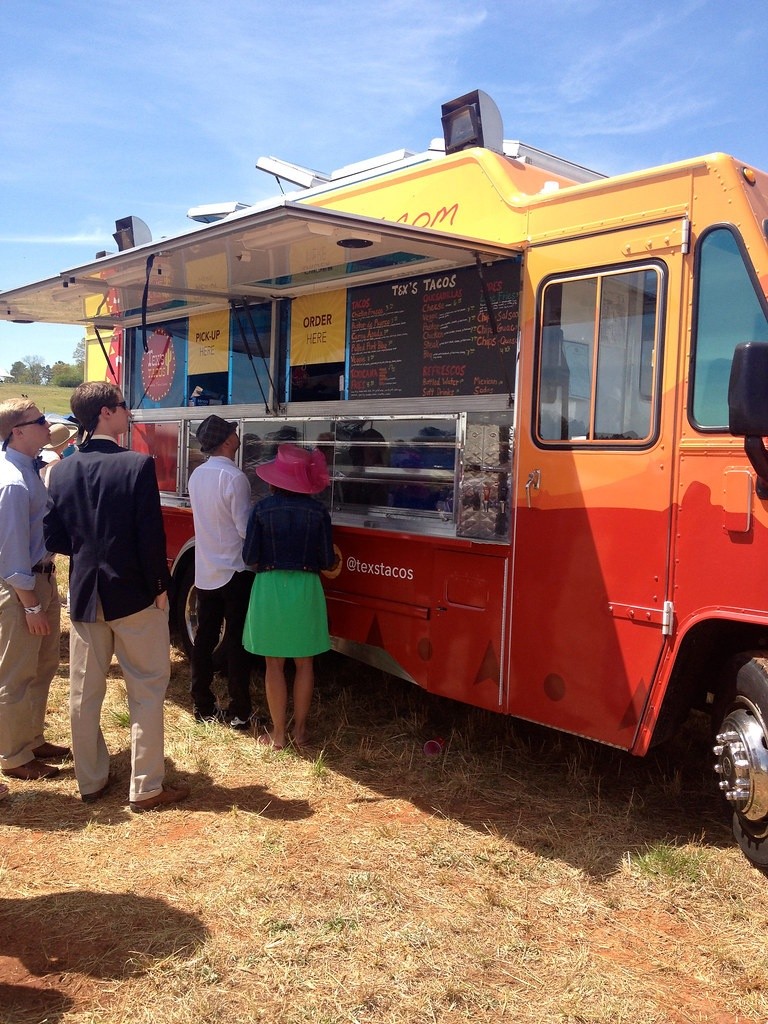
23, 602, 43, 614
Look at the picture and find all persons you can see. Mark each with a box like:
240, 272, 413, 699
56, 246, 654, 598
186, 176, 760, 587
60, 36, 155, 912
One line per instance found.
36, 424, 79, 490
188, 414, 272, 732
0, 396, 71, 780
234, 423, 499, 520
42, 380, 193, 814
241, 442, 337, 750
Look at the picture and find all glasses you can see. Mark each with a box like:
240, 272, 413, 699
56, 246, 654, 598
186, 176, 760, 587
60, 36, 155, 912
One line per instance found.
109, 401, 126, 409
14, 415, 45, 428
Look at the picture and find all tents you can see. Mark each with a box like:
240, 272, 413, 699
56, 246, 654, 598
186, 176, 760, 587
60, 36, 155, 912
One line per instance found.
41, 411, 87, 446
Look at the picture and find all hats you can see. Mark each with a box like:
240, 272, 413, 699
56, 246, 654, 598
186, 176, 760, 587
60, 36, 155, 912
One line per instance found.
256, 444, 326, 494
42, 423, 78, 449
196, 416, 238, 454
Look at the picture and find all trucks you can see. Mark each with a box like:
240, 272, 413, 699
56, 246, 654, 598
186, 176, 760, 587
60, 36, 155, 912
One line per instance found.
0, 85, 766, 872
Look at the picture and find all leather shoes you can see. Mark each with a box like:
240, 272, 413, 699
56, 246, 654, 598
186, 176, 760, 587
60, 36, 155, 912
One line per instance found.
2, 743, 71, 779
82, 774, 191, 814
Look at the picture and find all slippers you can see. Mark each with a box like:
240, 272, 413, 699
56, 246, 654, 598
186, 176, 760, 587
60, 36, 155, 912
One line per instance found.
258, 732, 316, 752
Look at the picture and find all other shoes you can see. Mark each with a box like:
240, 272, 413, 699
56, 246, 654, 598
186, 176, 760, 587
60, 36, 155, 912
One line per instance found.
193, 708, 253, 728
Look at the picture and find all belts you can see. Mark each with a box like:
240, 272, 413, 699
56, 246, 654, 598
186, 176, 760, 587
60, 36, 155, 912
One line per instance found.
30, 564, 56, 573
